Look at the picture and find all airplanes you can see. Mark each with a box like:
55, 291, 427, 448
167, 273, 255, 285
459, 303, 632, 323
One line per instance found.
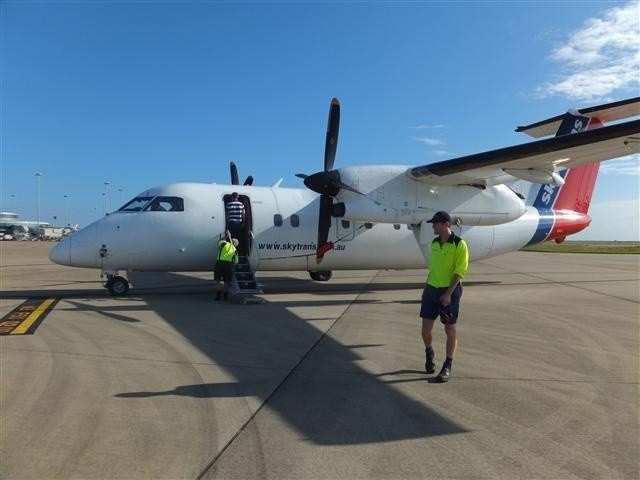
46, 96, 640, 297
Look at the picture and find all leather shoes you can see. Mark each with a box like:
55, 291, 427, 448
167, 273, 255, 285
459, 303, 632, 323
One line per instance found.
437, 362, 451, 382
214, 296, 227, 300
425, 351, 436, 373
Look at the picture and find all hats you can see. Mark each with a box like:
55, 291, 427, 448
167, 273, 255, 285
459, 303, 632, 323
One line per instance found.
426, 211, 451, 224
233, 238, 239, 247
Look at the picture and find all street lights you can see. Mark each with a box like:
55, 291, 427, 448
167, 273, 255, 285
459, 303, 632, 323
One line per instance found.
53, 178, 124, 226
35, 171, 43, 230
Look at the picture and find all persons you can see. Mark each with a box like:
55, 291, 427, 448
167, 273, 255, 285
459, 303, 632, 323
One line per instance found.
225, 192, 246, 250
420, 211, 469, 381
213, 228, 239, 301
